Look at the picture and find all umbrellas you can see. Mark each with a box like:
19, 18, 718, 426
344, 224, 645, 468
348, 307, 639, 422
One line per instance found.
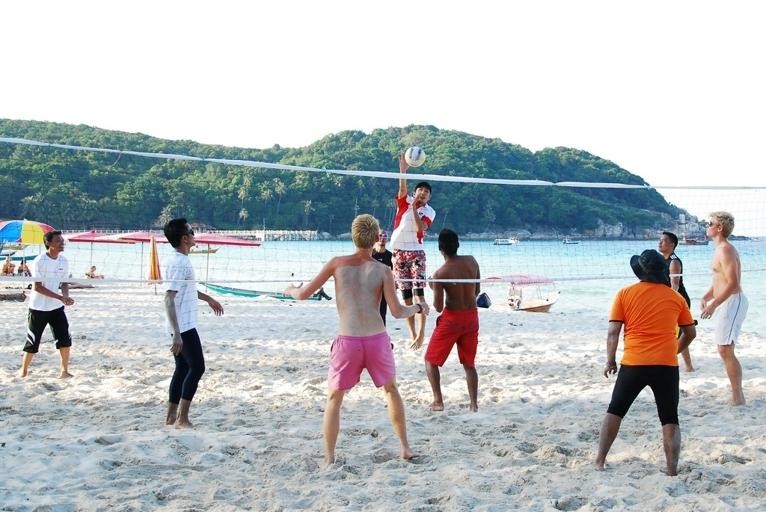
0, 218, 59, 279
163, 232, 261, 294
67, 229, 135, 282
144, 237, 165, 296
119, 226, 171, 285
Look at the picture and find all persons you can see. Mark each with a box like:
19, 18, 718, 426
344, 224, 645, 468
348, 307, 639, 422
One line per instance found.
162, 218, 224, 431
282, 214, 430, 469
18, 228, 75, 382
508, 281, 523, 311
593, 249, 696, 477
85, 263, 106, 281
369, 227, 399, 329
17, 260, 32, 278
389, 154, 435, 352
651, 228, 701, 377
424, 227, 484, 415
309, 288, 334, 301
698, 206, 752, 407
1, 256, 14, 277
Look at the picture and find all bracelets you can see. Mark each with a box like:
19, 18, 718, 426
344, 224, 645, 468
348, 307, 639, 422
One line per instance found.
605, 360, 617, 369
416, 304, 424, 315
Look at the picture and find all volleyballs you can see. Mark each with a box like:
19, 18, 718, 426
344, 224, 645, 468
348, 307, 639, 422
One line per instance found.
405, 147, 425, 167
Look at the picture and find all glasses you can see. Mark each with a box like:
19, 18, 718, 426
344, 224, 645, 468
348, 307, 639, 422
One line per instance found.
187, 228, 195, 235
708, 221, 715, 227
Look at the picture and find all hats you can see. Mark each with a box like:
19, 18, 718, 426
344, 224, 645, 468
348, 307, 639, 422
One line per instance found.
629, 248, 671, 284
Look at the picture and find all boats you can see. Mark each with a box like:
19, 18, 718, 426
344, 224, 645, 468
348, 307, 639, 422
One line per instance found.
492, 236, 521, 246
0, 250, 17, 256
0, 254, 38, 262
190, 246, 222, 254
199, 280, 332, 302
501, 290, 563, 312
679, 233, 709, 245
563, 235, 580, 244
